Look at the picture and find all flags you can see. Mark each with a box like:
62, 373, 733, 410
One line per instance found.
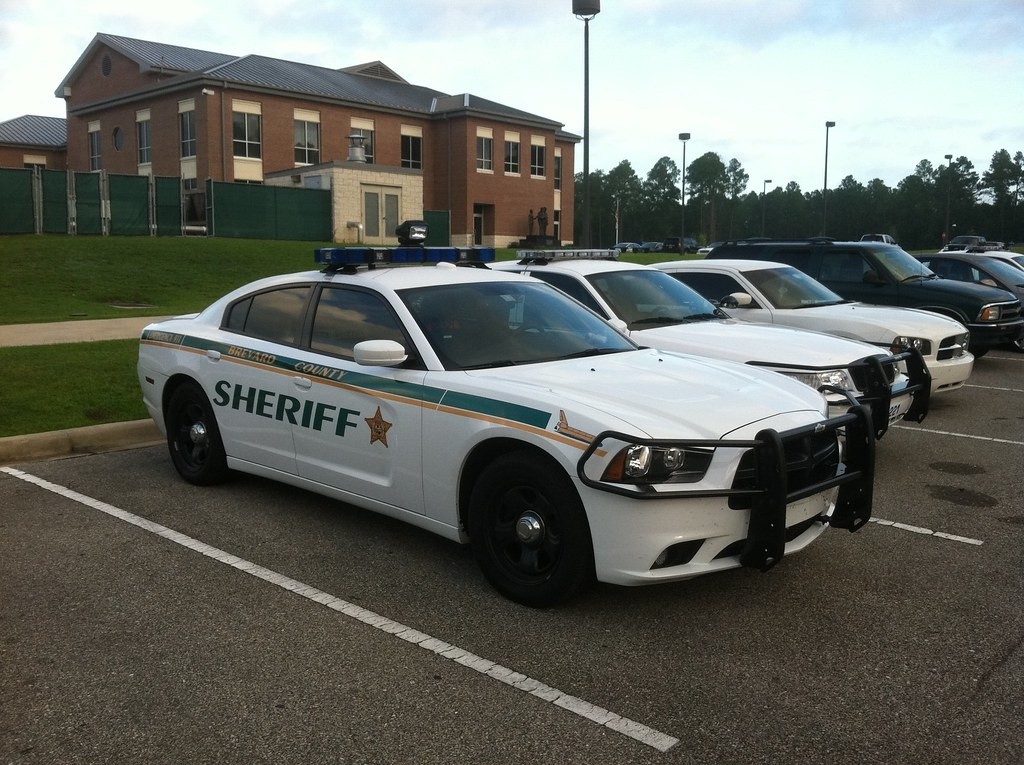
615, 208, 619, 229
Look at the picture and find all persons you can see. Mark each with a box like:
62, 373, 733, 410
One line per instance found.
475, 292, 508, 335
528, 209, 536, 234
536, 207, 548, 235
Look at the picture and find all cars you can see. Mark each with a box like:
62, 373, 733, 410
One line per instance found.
663, 237, 698, 254
923, 250, 1024, 287
633, 242, 663, 254
596, 259, 974, 395
607, 243, 642, 253
137, 245, 874, 607
943, 236, 986, 252
480, 248, 932, 440
697, 242, 724, 255
888, 253, 1024, 353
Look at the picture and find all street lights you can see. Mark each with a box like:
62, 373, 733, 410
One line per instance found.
762, 180, 772, 237
821, 122, 835, 237
679, 133, 690, 255
572, 0, 600, 249
945, 155, 952, 244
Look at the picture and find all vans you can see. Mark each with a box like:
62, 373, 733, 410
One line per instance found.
860, 234, 898, 245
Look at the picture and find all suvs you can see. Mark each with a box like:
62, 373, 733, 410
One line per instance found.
701, 239, 1024, 359
